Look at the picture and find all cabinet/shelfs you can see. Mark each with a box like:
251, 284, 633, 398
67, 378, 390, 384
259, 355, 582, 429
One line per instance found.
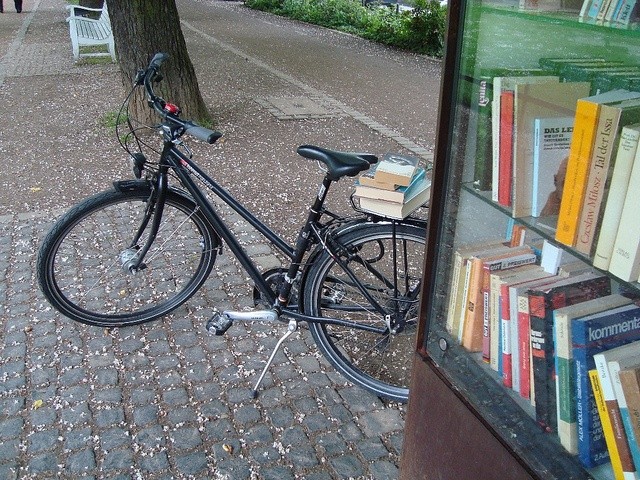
397, 2, 640, 480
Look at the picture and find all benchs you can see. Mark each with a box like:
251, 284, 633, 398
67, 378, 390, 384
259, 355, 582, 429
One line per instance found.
65, 0, 119, 63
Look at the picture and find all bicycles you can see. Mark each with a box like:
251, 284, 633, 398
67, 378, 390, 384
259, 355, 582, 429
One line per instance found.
35, 52, 429, 403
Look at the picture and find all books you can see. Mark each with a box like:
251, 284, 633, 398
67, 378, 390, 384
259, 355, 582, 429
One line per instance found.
374, 152, 419, 187
353, 180, 431, 220
572, 99, 640, 256
517, 0, 640, 28
535, 55, 640, 118
592, 123, 640, 271
559, 248, 610, 285
473, 68, 534, 217
358, 162, 418, 190
443, 285, 639, 480
554, 89, 640, 249
607, 139, 640, 283
353, 168, 425, 203
532, 118, 575, 217
451, 218, 560, 285
